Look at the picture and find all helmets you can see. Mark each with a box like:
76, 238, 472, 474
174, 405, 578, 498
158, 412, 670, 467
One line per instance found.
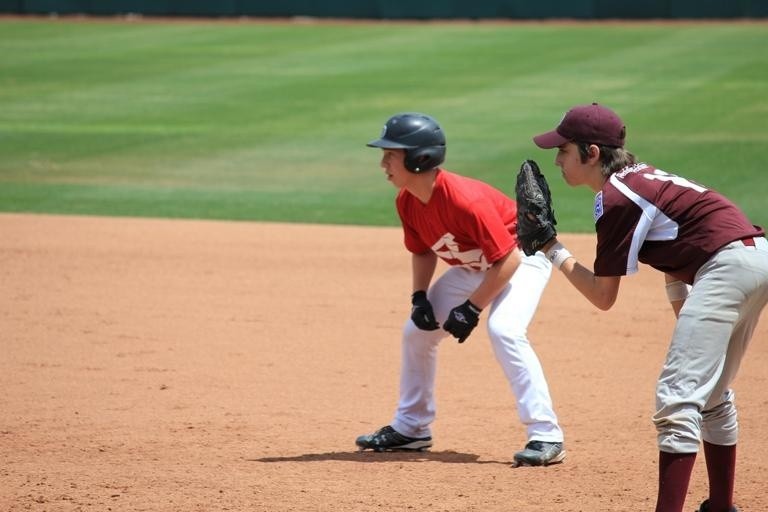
366, 113, 446, 174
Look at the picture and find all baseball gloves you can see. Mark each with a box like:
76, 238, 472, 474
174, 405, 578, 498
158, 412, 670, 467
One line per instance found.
517, 159, 558, 256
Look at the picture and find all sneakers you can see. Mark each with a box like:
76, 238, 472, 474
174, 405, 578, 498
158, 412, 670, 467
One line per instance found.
514, 441, 567, 467
356, 425, 432, 452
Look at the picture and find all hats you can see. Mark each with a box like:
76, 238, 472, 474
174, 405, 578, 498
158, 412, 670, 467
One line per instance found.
532, 104, 627, 148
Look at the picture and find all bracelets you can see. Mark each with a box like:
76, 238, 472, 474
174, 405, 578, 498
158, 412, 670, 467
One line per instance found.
543, 236, 574, 273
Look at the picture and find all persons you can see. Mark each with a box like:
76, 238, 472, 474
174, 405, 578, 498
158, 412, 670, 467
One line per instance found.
509, 98, 767, 512
355, 111, 566, 473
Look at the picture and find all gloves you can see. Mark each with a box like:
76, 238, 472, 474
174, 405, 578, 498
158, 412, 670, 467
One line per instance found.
410, 288, 440, 332
443, 299, 483, 344
